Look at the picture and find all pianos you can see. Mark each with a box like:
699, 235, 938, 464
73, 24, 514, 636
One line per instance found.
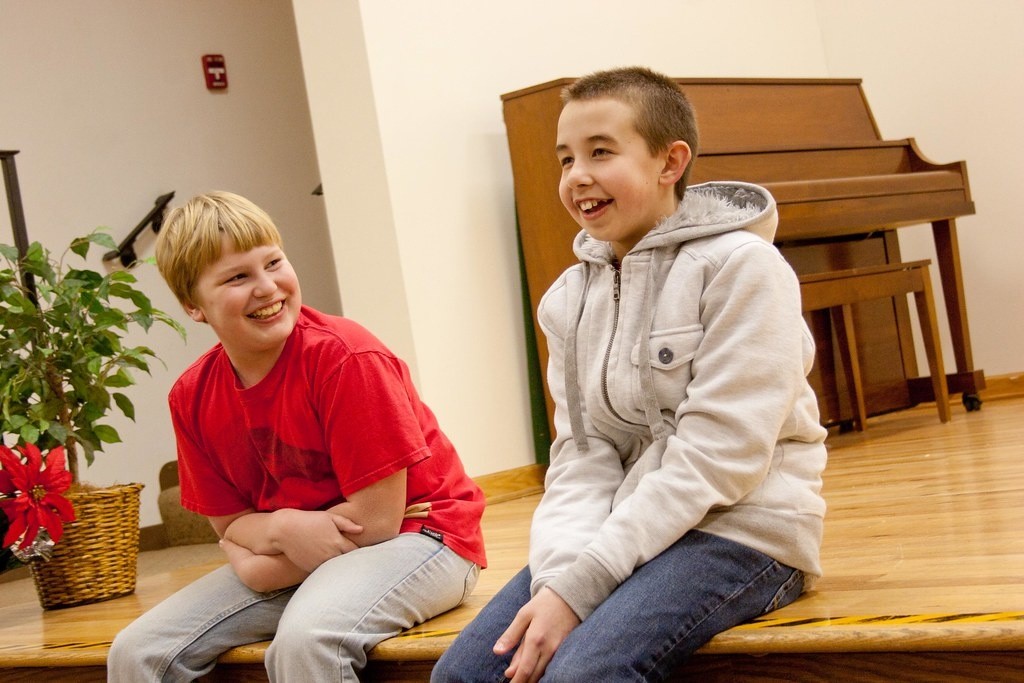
497, 76, 989, 452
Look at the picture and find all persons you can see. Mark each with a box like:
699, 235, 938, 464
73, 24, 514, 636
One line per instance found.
429, 64, 828, 683
106, 190, 488, 682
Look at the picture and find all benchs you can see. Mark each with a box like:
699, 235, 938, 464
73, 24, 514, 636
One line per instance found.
796, 259, 954, 433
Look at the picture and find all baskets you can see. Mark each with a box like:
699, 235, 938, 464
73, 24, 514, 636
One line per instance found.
1, 483, 146, 609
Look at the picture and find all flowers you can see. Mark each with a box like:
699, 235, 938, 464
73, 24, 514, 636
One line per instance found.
0, 441, 76, 550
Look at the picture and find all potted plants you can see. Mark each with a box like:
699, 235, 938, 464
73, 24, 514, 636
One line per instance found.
0, 224, 189, 611
